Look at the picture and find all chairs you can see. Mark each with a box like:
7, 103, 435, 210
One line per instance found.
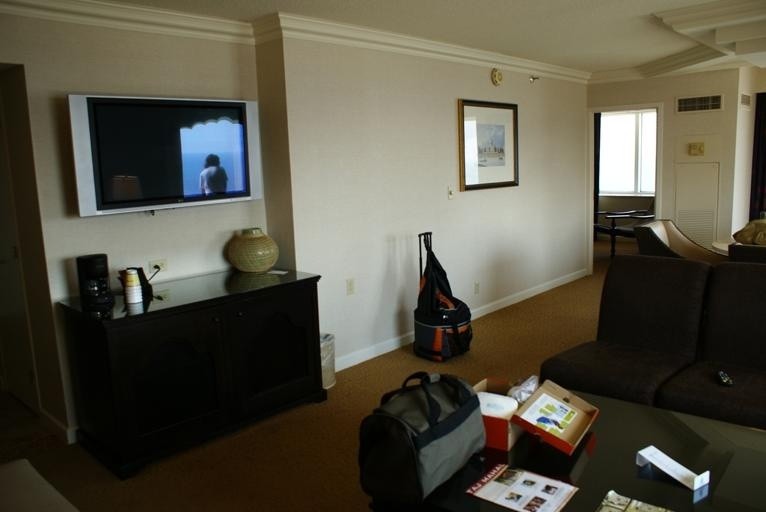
595, 194, 657, 257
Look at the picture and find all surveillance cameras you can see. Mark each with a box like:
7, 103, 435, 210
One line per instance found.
489, 66, 506, 89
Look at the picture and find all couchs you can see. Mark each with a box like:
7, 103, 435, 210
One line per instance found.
635, 218, 733, 264
541, 257, 766, 431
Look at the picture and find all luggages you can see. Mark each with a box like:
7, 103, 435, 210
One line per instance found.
413, 231, 472, 364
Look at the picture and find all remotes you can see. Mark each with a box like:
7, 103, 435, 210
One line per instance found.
634, 445, 714, 493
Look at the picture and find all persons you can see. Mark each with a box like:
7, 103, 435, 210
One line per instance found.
199, 153, 227, 198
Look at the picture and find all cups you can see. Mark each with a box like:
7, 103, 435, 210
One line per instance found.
125, 269, 143, 304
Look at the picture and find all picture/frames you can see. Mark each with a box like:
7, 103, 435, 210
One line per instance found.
458, 98, 520, 192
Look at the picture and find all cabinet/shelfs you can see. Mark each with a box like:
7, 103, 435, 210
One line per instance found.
54, 265, 328, 478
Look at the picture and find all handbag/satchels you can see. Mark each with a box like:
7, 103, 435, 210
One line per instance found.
357, 370, 486, 504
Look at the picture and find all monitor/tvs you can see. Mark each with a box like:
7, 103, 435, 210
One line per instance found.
67, 94, 265, 218
